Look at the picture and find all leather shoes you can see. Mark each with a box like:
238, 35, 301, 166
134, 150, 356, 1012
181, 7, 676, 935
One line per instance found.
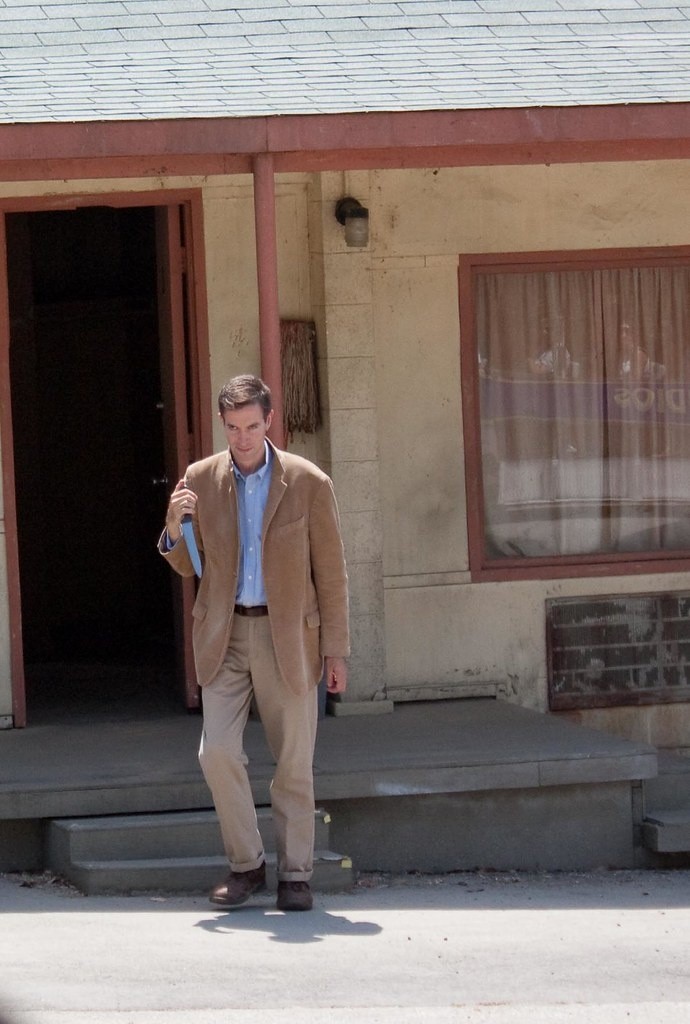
277, 880, 312, 910
210, 862, 266, 903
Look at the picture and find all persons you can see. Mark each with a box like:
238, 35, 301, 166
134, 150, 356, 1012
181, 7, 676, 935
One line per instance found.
525, 312, 570, 375
608, 311, 668, 383
157, 375, 351, 911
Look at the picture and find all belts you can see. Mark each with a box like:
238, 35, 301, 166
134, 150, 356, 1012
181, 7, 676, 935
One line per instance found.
233, 605, 267, 618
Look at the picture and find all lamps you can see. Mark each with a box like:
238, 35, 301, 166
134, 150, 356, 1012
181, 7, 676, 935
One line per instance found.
336, 195, 369, 247
